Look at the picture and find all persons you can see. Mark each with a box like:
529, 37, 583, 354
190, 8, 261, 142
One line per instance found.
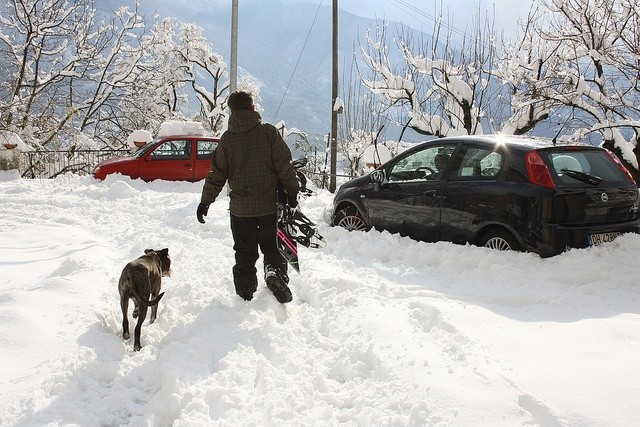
196, 91, 299, 303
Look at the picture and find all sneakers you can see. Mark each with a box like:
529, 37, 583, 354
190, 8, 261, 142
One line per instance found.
265, 270, 292, 303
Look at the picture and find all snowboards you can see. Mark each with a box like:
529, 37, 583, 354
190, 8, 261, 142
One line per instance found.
275, 183, 300, 274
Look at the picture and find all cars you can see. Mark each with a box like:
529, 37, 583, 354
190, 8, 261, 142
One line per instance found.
93, 135, 221, 183
330, 133, 636, 258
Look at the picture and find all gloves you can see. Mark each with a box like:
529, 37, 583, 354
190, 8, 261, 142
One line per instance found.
288, 191, 300, 207
197, 203, 209, 223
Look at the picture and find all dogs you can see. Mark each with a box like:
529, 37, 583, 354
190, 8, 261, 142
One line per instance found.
117, 247, 172, 351
